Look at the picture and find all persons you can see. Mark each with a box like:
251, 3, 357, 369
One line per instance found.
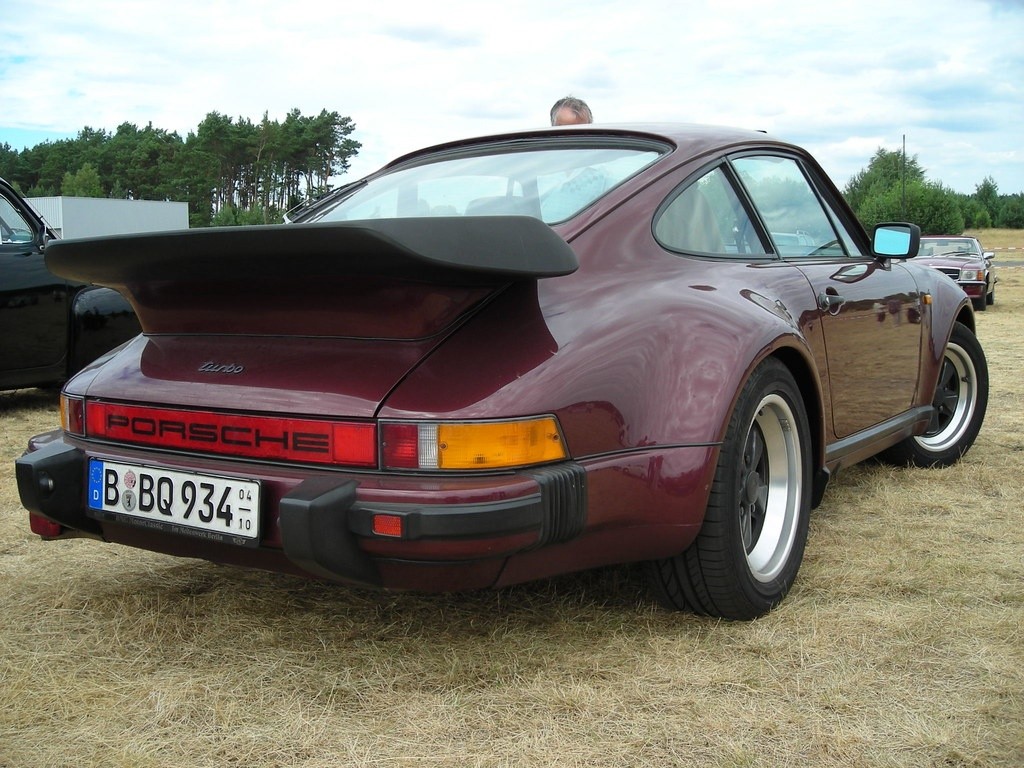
549, 96, 594, 126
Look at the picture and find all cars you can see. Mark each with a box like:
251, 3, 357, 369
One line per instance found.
0, 178, 142, 394
913, 234, 997, 311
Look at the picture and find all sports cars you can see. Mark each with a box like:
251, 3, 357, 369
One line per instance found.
15, 124, 987, 624
